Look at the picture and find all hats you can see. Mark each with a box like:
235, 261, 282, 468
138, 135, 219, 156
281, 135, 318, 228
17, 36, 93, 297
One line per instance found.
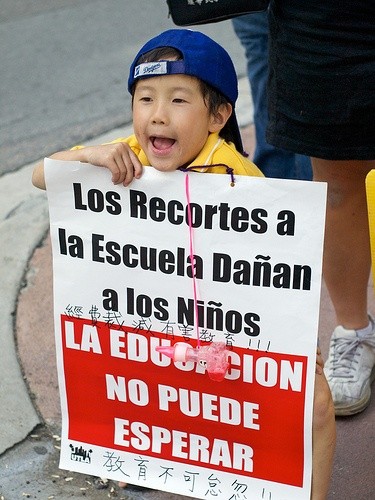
128, 29, 244, 157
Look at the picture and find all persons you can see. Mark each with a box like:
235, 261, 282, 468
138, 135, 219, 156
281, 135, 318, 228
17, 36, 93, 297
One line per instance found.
225, 0, 375, 418
30, 28, 337, 500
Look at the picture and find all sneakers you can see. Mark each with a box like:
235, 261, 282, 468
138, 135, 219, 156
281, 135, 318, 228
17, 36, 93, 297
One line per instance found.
323, 311, 375, 415
